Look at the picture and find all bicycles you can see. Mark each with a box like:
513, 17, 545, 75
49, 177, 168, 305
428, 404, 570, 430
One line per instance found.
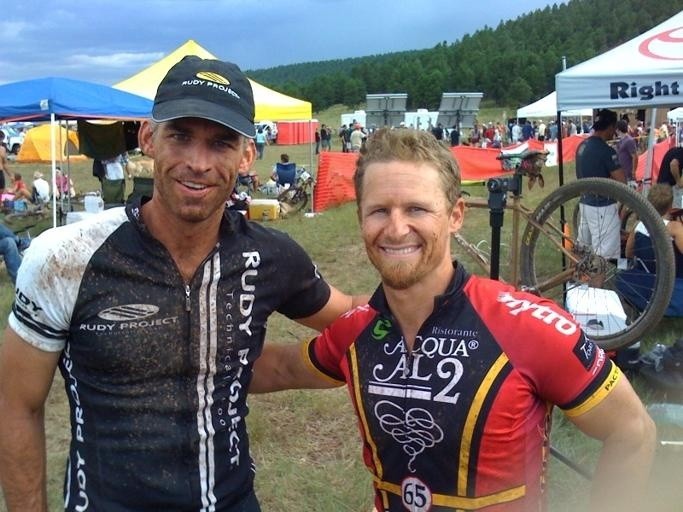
450, 136, 677, 357
276, 166, 317, 215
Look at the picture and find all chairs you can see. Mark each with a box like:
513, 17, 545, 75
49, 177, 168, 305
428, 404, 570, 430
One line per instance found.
629, 229, 681, 279
275, 159, 294, 187
236, 172, 255, 195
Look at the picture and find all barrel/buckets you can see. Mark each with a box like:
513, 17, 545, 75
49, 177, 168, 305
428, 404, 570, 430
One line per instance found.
84, 195, 105, 213
3, 197, 14, 215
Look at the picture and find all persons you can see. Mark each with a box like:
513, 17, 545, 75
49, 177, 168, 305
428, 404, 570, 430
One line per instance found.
248, 129, 658, 512
0, 53, 374, 512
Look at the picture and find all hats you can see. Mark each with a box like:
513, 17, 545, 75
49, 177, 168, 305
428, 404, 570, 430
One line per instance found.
151, 55, 257, 140
591, 110, 617, 129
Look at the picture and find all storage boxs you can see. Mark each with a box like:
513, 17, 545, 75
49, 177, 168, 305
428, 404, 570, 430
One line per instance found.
248, 199, 278, 221
561, 282, 629, 362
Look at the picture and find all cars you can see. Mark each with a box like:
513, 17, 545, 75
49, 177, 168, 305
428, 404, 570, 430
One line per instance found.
0, 122, 36, 155
